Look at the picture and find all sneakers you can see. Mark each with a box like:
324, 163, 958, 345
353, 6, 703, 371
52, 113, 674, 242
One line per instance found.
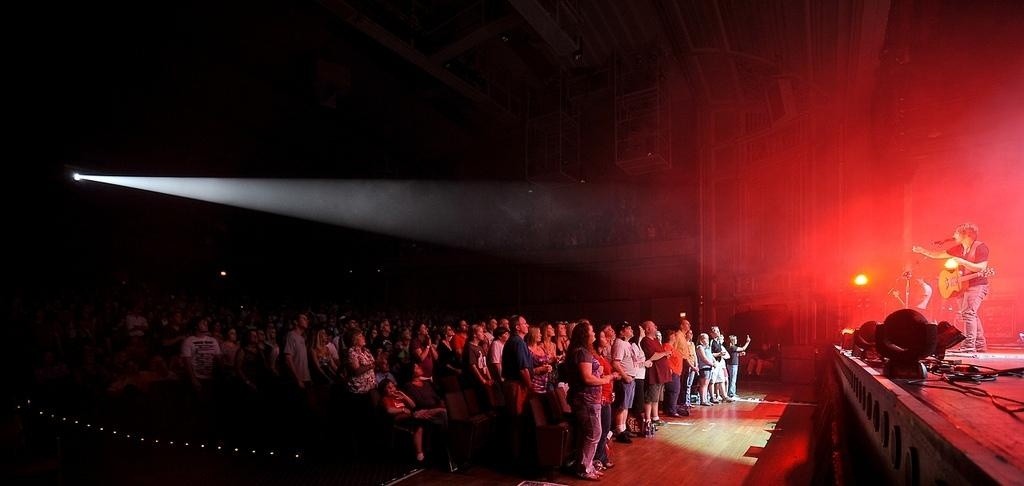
616, 392, 739, 444
976, 346, 988, 352
578, 458, 615, 482
948, 347, 977, 353
415, 457, 434, 470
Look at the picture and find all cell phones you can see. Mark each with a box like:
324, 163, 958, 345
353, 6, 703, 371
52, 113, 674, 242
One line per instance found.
747, 335, 749, 339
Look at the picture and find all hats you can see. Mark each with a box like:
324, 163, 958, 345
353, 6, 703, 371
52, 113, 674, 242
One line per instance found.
615, 320, 631, 331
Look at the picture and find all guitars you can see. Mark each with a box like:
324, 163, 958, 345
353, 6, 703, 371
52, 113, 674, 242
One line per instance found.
938, 271, 991, 298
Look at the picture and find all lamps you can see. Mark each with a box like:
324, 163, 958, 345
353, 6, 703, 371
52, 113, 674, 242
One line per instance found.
852, 320, 888, 364
873, 307, 940, 378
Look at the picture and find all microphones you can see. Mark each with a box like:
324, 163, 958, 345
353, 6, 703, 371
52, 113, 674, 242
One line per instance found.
934, 237, 955, 244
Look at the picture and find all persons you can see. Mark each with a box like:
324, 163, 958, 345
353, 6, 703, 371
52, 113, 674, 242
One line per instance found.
912, 220, 994, 359
0, 275, 782, 481
912, 274, 933, 315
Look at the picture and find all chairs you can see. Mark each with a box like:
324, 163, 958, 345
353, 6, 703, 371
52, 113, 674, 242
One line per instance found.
294, 362, 579, 475
